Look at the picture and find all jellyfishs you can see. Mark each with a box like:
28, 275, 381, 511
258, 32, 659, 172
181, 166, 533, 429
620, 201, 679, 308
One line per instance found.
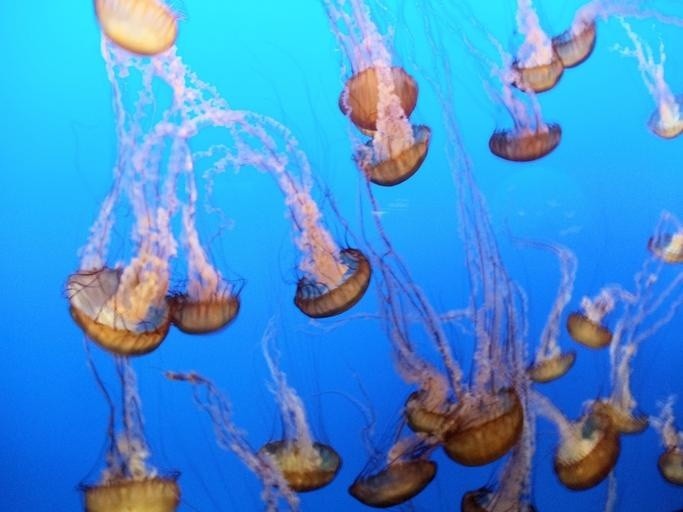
60, 0, 683, 512
